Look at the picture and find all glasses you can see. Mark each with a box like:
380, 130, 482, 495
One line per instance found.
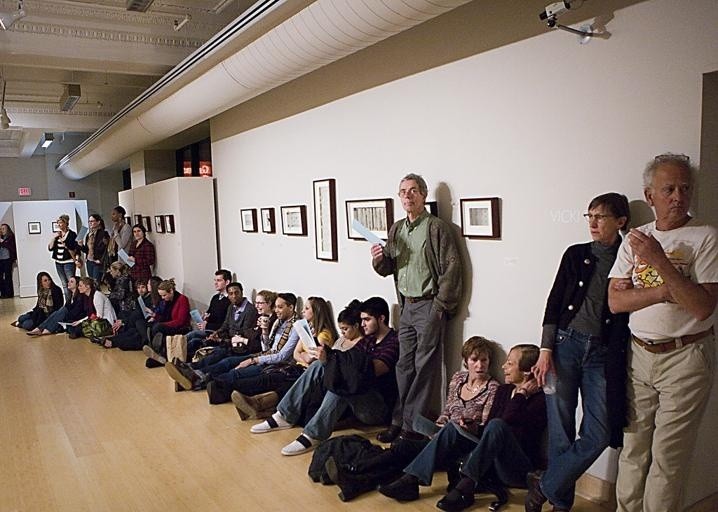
584, 214, 607, 223
253, 301, 263, 305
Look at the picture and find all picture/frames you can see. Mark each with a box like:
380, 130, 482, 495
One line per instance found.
27, 221, 42, 236
260, 206, 277, 235
423, 200, 439, 217
312, 178, 340, 264
459, 194, 503, 241
344, 198, 396, 242
51, 221, 62, 233
240, 207, 258, 234
280, 204, 309, 238
118, 213, 175, 236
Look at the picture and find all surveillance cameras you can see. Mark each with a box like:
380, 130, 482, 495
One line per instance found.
539, 0, 572, 23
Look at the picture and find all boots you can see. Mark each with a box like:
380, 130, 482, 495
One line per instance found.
230, 389, 280, 420
325, 450, 397, 502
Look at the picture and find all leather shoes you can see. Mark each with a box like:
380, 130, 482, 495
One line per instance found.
10, 321, 52, 336
90, 334, 109, 348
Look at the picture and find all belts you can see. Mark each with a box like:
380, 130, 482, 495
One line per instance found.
405, 294, 434, 304
632, 328, 714, 354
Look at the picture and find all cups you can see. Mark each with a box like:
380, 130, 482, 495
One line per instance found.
541, 372, 557, 394
260, 316, 269, 328
632, 228, 654, 239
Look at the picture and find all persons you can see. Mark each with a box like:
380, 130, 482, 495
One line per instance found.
605, 153, 718, 512
323, 336, 547, 512
523, 193, 631, 512
371, 175, 463, 448
11, 206, 157, 350
143, 269, 400, 457
0, 224, 17, 299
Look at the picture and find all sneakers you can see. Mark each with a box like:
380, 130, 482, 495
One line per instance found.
141, 345, 166, 368
281, 431, 327, 456
164, 357, 231, 404
377, 478, 421, 501
250, 413, 295, 433
377, 425, 424, 448
436, 472, 571, 511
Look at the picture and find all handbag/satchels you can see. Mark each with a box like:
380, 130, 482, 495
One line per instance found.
82, 318, 114, 338
232, 342, 251, 355
191, 346, 215, 362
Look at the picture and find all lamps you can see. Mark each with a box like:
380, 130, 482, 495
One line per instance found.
38, 130, 56, 148
56, 64, 83, 114
0, 0, 27, 32
